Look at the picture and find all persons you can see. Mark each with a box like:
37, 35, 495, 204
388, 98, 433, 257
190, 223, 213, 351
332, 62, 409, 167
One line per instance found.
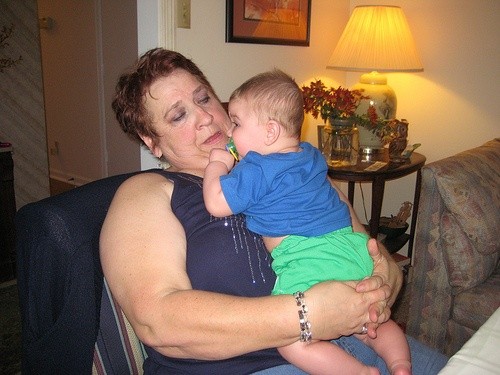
203, 67, 413, 374
99, 46, 450, 374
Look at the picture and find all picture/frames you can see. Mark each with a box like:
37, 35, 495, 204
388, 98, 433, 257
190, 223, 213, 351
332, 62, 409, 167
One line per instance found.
225, 0, 311, 47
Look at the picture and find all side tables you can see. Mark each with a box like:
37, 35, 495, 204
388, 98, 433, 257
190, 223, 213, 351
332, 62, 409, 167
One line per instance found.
327, 152, 426, 277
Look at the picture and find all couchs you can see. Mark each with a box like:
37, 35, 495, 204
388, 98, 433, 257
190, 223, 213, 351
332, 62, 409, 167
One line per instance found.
408, 137, 500, 358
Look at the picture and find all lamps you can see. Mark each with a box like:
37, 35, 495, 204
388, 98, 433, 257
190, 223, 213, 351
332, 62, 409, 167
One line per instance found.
325, 4, 424, 151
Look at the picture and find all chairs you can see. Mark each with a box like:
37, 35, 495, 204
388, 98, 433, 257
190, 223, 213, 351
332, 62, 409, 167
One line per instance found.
19, 170, 155, 375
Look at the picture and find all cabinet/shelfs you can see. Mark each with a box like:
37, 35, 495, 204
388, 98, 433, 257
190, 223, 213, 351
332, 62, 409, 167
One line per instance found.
0, 153, 19, 288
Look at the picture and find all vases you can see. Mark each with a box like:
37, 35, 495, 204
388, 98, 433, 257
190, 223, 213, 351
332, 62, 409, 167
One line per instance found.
317, 119, 358, 167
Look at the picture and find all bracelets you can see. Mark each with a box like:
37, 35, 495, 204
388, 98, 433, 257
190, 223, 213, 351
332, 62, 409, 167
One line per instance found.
293, 291, 312, 344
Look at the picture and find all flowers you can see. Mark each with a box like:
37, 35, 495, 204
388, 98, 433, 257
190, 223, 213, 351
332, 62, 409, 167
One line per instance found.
304, 79, 379, 129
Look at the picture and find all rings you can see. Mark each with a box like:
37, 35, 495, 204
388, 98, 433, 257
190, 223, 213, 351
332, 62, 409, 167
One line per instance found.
361, 325, 367, 334
